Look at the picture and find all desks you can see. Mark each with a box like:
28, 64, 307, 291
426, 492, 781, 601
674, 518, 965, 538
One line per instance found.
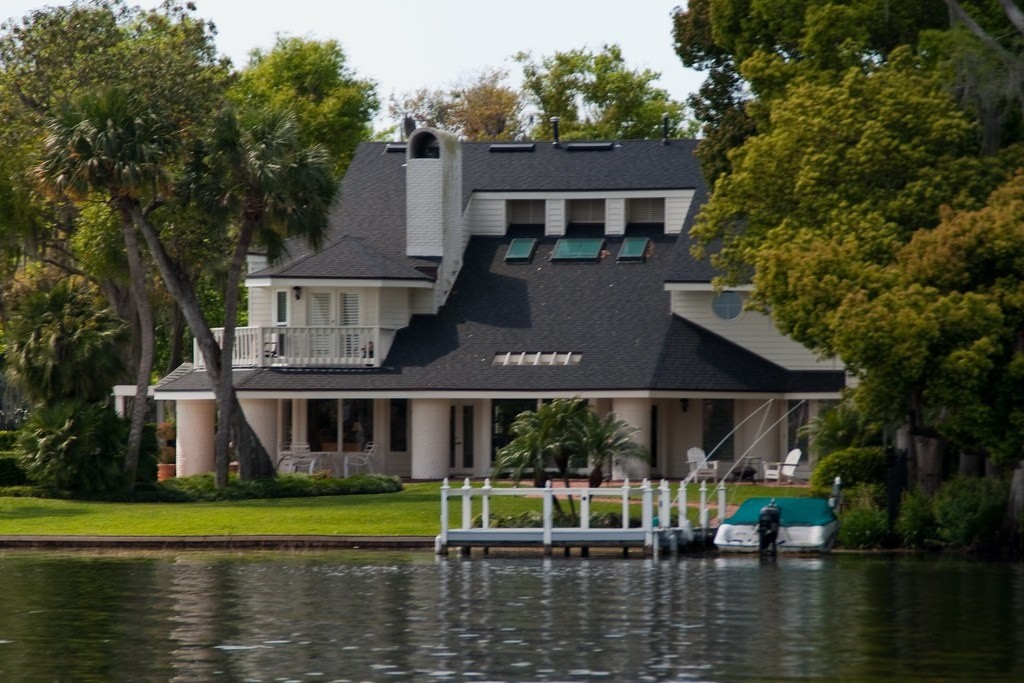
277, 451, 339, 475
737, 455, 762, 482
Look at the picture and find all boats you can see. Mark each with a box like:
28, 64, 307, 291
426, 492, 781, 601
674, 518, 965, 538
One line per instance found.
713, 496, 838, 556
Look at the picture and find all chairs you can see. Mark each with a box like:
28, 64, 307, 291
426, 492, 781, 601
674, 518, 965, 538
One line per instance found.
330, 440, 377, 477
761, 448, 803, 484
256, 342, 279, 359
290, 441, 322, 473
686, 447, 719, 483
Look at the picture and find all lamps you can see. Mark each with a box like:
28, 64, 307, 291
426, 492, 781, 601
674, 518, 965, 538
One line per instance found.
680, 399, 688, 413
292, 285, 302, 300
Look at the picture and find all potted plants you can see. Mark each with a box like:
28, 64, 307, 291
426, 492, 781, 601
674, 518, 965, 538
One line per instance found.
154, 423, 176, 481
227, 446, 239, 472
